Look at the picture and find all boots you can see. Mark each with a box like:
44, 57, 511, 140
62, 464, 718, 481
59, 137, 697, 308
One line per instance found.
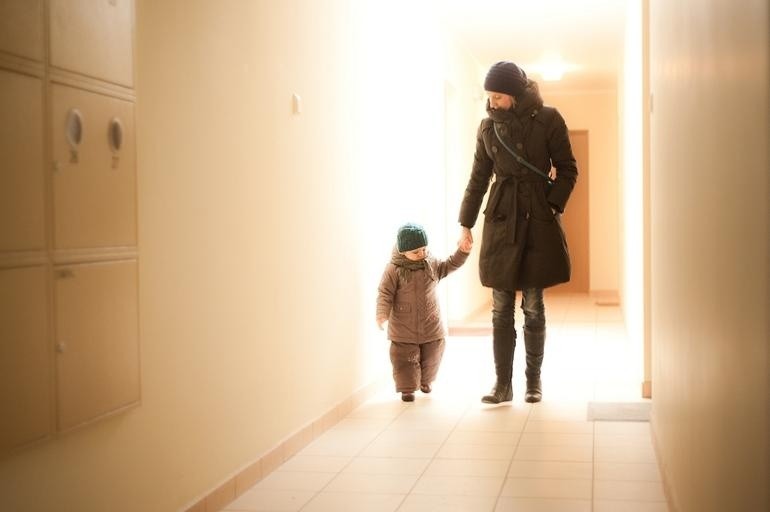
523, 325, 545, 403
481, 327, 517, 405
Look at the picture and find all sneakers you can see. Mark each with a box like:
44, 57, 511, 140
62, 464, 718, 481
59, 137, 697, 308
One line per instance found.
420, 383, 431, 393
402, 390, 415, 402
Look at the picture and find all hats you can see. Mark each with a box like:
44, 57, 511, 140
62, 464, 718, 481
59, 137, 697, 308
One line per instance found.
397, 226, 428, 253
485, 62, 527, 96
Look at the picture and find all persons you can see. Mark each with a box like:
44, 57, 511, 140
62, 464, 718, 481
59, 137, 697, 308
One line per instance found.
457, 61, 578, 406
376, 223, 473, 403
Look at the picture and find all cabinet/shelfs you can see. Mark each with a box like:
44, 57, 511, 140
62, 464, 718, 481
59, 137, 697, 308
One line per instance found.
1, 0, 144, 466
